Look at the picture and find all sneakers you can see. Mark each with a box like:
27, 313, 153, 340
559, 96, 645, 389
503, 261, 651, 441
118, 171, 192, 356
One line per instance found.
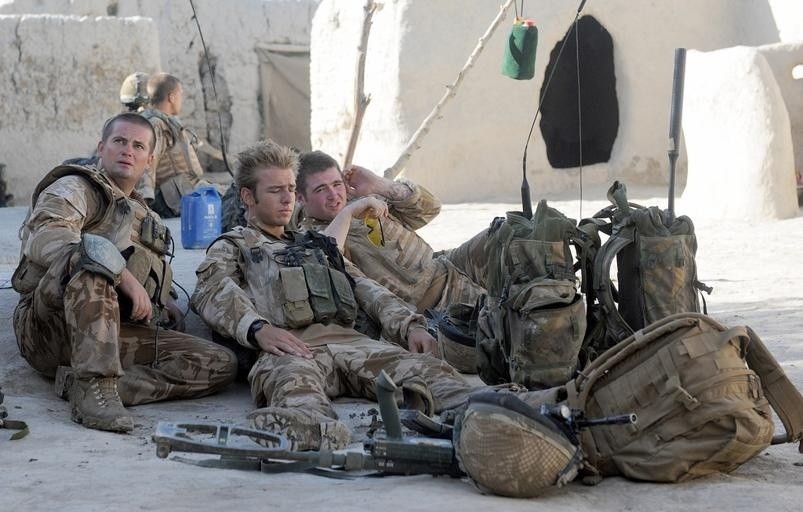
53, 365, 74, 401
245, 407, 349, 453
71, 377, 135, 432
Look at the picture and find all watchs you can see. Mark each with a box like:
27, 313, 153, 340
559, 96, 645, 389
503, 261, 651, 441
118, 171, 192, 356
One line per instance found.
248, 321, 267, 345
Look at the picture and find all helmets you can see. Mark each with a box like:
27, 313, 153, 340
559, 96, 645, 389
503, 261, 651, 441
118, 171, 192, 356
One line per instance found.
119, 73, 150, 106
451, 391, 583, 499
439, 307, 476, 374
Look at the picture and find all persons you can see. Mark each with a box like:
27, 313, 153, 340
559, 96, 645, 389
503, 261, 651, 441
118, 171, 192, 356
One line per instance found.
138, 73, 232, 218
288, 151, 496, 374
121, 71, 150, 110
11, 113, 238, 430
188, 137, 564, 452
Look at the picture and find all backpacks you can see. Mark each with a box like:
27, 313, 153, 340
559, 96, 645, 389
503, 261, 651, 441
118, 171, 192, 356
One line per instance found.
578, 180, 713, 350
568, 311, 803, 486
221, 183, 247, 233
476, 199, 599, 390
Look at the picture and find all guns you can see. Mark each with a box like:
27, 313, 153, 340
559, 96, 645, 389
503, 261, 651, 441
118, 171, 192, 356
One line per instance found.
399, 404, 638, 446
363, 369, 468, 479
